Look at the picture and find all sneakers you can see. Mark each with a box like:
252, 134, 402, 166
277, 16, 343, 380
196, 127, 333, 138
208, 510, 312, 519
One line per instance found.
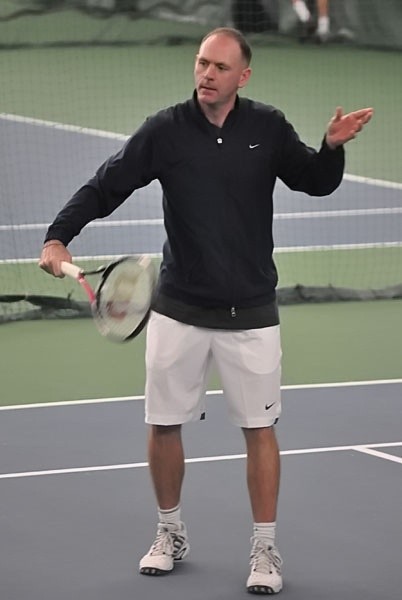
246, 535, 284, 594
139, 521, 190, 576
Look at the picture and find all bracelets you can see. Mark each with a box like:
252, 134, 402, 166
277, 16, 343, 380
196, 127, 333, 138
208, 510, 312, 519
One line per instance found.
41, 243, 63, 250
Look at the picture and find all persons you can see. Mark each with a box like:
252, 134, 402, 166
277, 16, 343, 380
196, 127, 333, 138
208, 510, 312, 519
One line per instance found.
39, 28, 374, 595
